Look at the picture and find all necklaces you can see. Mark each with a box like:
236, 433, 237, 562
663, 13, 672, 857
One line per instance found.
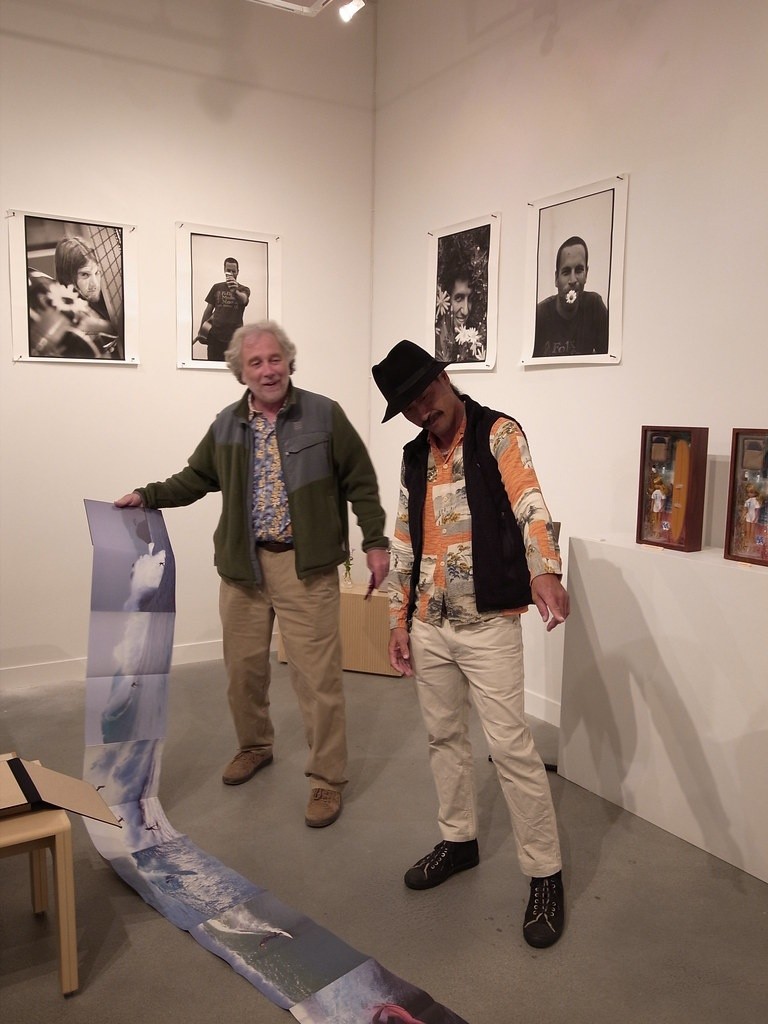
430, 443, 456, 467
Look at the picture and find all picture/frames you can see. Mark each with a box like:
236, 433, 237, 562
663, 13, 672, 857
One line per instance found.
518, 173, 630, 366
8, 208, 140, 364
427, 213, 501, 370
175, 220, 280, 369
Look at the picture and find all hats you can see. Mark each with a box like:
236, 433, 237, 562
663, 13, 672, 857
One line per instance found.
372, 340, 455, 424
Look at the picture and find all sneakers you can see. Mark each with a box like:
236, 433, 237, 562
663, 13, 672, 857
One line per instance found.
523, 869, 564, 950
305, 788, 341, 828
222, 747, 273, 785
404, 838, 479, 891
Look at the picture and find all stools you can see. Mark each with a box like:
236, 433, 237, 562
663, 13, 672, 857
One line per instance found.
0, 760, 79, 993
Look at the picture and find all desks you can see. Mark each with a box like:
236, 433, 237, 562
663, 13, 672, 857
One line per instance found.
278, 584, 411, 677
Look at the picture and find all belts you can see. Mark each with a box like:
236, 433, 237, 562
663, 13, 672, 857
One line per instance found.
256, 540, 294, 553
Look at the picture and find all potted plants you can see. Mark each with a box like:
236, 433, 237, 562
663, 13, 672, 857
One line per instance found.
341, 548, 354, 588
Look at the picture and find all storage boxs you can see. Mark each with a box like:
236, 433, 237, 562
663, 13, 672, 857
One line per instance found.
724, 428, 768, 567
636, 425, 709, 552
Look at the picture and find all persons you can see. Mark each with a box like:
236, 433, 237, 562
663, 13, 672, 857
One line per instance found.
370, 339, 570, 948
537, 237, 607, 356
200, 257, 251, 361
651, 478, 666, 538
54, 236, 100, 303
115, 320, 390, 829
435, 259, 475, 362
740, 484, 762, 546
